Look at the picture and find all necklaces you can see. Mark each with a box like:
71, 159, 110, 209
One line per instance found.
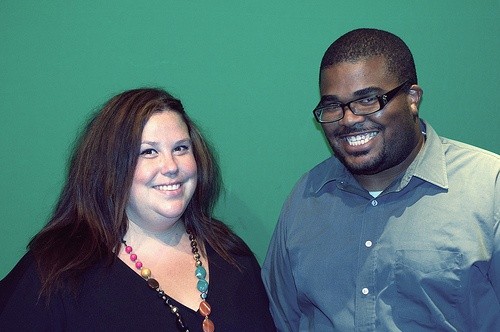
122, 222, 215, 332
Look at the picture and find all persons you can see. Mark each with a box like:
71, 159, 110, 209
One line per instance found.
260, 29, 500, 332
0, 87, 277, 332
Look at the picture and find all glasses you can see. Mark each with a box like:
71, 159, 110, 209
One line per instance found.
313, 80, 413, 124
